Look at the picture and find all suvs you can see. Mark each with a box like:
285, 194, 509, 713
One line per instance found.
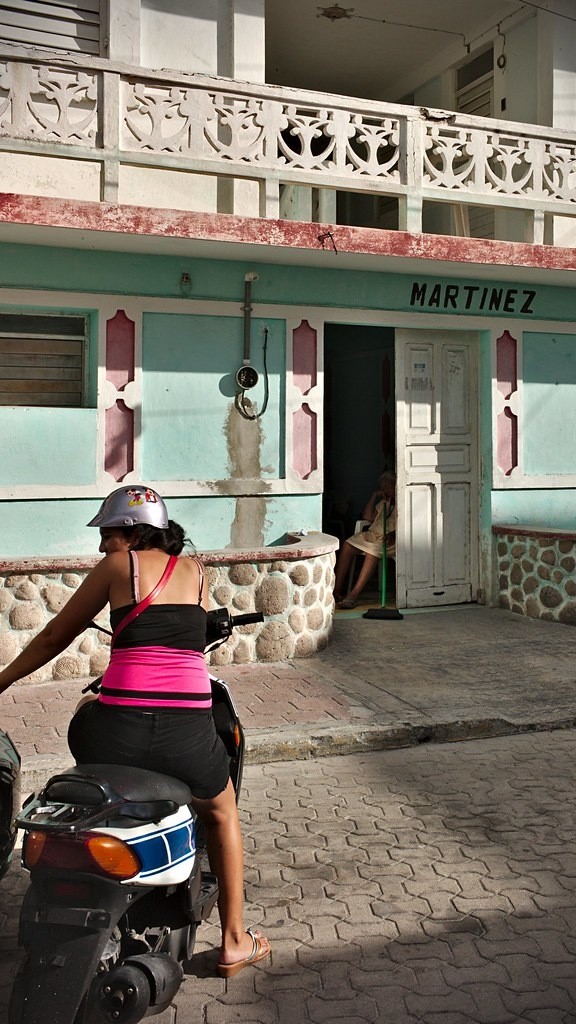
7, 608, 265, 1023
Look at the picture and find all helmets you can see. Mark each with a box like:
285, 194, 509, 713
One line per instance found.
86, 485, 168, 529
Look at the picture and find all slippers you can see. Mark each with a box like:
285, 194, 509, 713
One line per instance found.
216, 927, 271, 977
337, 598, 359, 609
334, 595, 344, 603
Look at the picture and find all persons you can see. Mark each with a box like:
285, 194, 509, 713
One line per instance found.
0, 486, 271, 976
333, 468, 396, 609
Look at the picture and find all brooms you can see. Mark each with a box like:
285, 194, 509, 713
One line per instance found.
361, 502, 404, 621
